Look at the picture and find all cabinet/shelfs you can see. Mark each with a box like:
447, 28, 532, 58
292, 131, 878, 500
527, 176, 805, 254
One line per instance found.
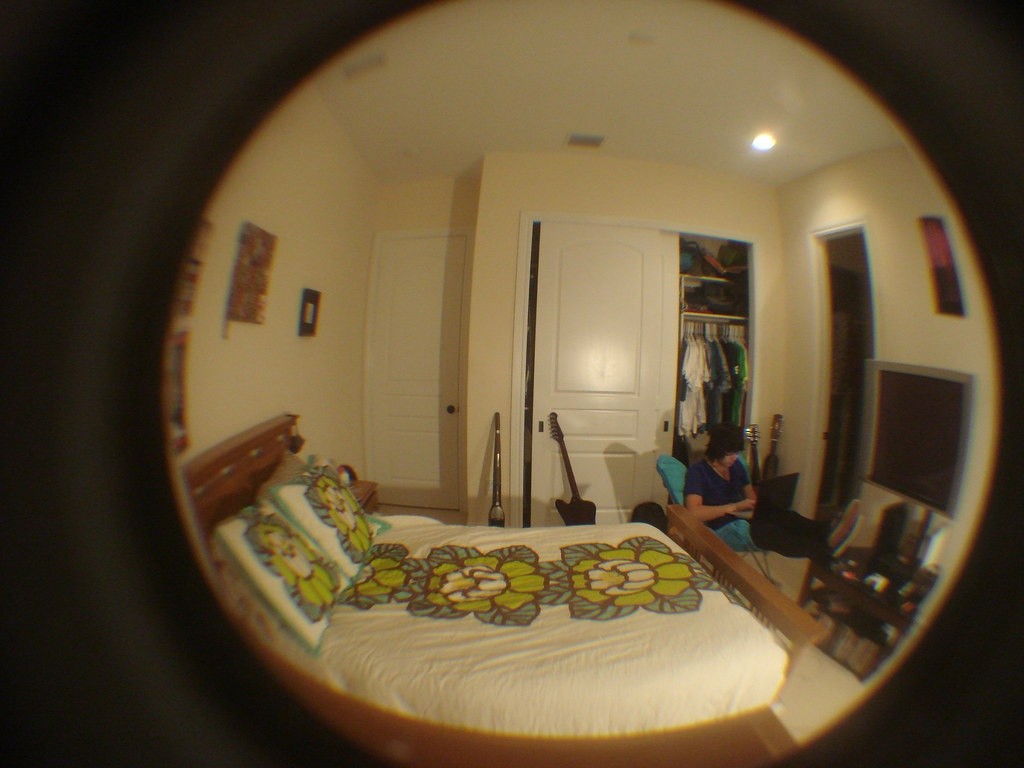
798, 546, 937, 685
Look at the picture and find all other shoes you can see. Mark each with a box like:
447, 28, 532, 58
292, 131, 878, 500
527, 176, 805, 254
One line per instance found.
832, 516, 864, 560
825, 499, 860, 548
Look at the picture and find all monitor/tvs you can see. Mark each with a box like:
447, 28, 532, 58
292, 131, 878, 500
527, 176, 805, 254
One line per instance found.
852, 360, 976, 521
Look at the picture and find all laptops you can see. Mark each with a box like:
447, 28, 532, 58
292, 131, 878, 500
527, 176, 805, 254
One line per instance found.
727, 472, 799, 522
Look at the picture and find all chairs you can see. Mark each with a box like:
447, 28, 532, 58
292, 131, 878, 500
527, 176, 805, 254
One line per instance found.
653, 450, 800, 582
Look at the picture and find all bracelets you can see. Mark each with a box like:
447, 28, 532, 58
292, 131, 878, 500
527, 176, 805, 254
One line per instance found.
730, 502, 736, 511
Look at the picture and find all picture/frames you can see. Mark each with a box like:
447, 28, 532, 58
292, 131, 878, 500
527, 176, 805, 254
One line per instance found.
298, 287, 320, 337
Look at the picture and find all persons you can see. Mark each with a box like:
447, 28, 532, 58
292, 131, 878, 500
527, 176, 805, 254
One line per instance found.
684, 421, 864, 558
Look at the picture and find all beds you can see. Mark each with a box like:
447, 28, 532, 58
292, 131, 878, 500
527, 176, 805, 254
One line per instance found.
178, 407, 829, 742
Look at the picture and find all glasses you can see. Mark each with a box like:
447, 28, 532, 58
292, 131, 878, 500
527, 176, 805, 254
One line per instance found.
725, 451, 740, 457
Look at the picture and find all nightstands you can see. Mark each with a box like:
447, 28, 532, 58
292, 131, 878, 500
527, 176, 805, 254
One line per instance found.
347, 478, 378, 515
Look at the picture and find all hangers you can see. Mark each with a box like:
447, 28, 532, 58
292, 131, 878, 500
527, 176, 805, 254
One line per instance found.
684, 319, 747, 346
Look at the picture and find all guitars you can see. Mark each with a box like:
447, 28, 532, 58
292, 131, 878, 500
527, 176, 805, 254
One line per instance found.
744, 424, 761, 483
547, 411, 596, 526
763, 414, 784, 480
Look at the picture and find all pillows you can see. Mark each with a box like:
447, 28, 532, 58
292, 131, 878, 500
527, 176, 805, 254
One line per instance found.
206, 498, 356, 654
268, 451, 392, 584
257, 452, 308, 509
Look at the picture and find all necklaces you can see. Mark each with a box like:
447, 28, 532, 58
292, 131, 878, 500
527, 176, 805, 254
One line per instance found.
706, 458, 724, 479
